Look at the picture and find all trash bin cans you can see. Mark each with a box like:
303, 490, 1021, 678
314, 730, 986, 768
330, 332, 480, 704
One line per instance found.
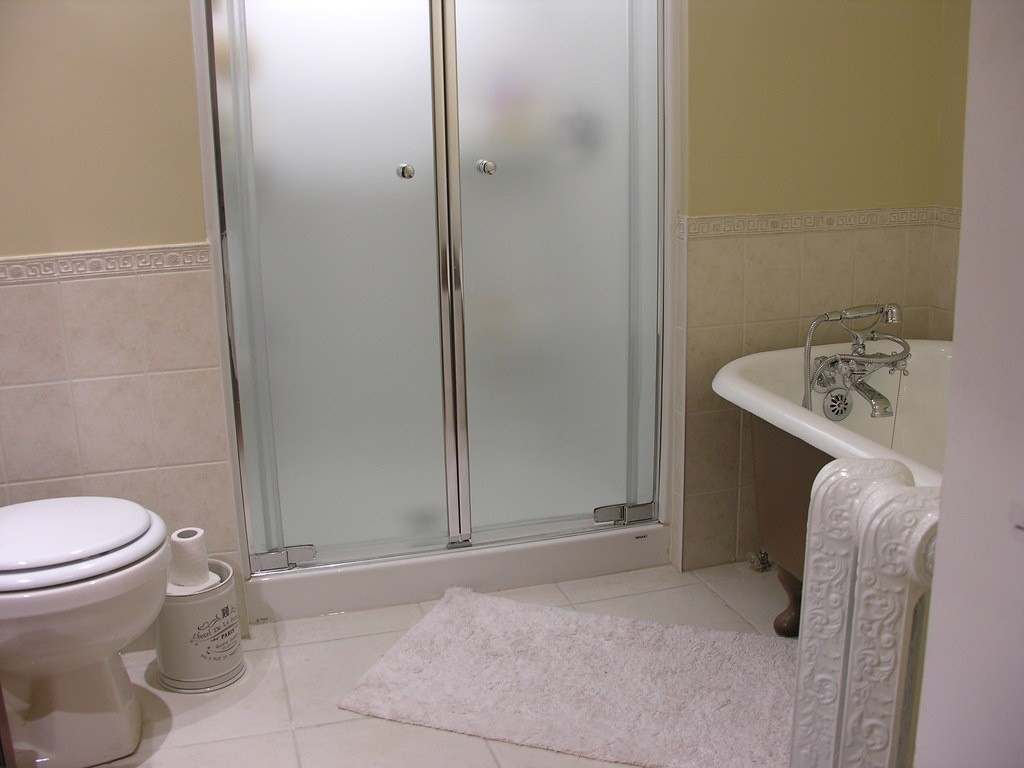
154, 558, 248, 694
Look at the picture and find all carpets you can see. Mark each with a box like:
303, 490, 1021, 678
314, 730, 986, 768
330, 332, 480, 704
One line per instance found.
338, 585, 797, 768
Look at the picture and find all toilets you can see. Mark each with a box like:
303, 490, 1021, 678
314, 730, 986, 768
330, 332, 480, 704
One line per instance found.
0, 496, 169, 768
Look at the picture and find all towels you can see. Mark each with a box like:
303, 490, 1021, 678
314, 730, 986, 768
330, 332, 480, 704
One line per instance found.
335, 584, 797, 766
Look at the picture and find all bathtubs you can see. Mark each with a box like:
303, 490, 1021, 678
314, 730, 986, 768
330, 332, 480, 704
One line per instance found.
711, 341, 956, 636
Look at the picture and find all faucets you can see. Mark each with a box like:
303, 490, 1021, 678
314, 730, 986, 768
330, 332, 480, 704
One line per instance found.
855, 382, 893, 418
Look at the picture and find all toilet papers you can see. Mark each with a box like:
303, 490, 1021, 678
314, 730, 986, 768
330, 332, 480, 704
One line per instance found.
168, 528, 209, 586
